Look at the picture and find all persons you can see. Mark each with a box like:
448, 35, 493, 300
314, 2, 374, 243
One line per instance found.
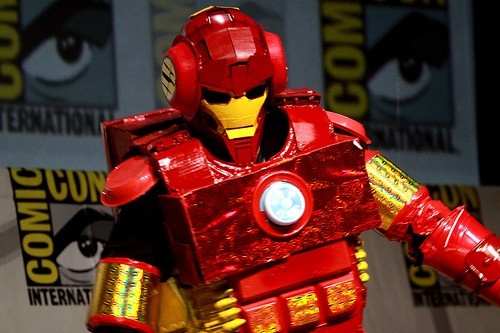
86, 6, 500, 333
